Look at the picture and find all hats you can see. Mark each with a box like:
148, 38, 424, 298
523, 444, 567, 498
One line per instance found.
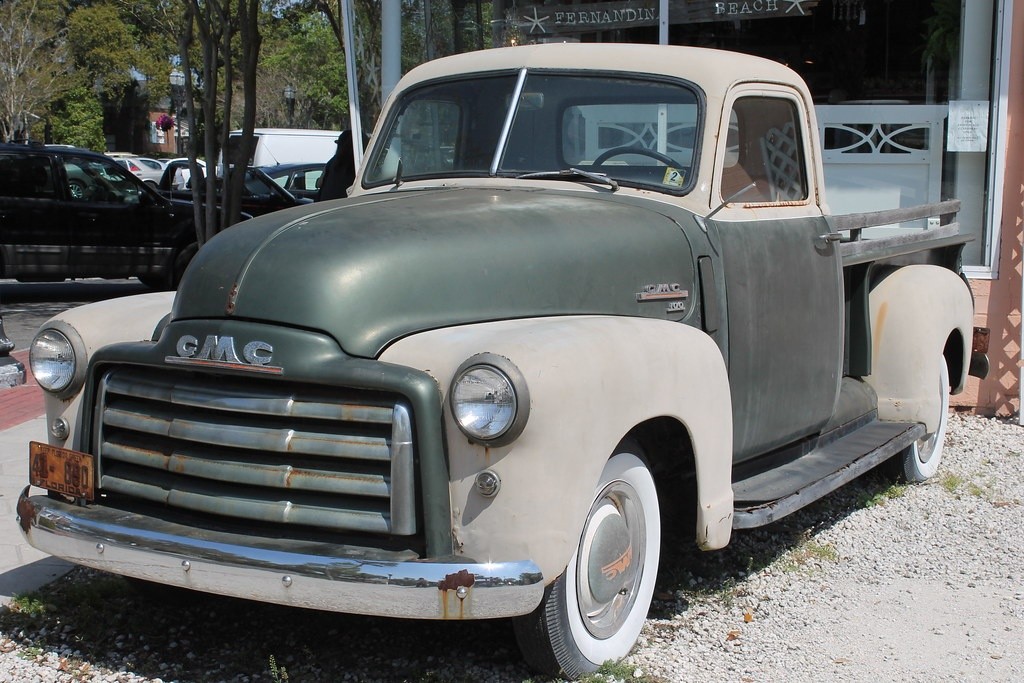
335, 130, 369, 146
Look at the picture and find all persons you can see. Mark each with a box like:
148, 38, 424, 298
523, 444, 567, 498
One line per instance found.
314, 130, 370, 201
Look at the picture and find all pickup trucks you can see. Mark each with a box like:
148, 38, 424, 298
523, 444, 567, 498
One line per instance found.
16, 42, 990, 683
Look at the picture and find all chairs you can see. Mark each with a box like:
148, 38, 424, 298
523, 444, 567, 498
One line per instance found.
720, 134, 777, 202
290, 176, 305, 190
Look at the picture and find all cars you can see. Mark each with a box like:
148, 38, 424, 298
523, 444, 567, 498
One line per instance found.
0, 124, 403, 294
